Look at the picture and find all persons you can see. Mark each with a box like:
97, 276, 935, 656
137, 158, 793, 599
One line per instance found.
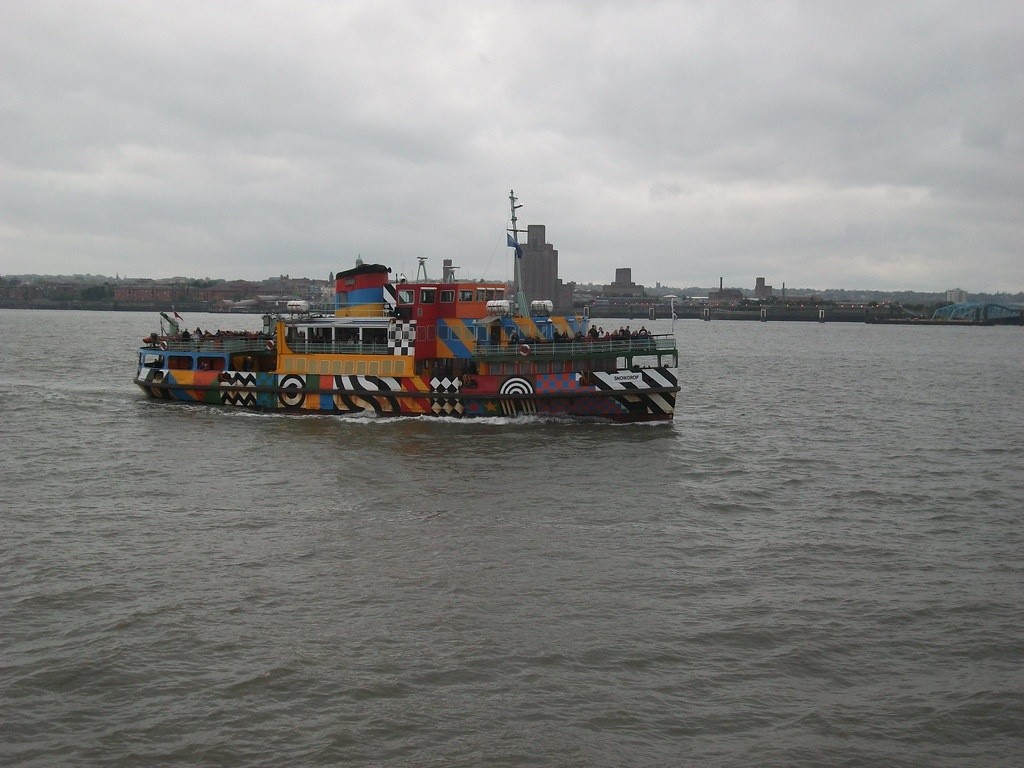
182, 326, 271, 341
299, 331, 387, 341
510, 325, 652, 342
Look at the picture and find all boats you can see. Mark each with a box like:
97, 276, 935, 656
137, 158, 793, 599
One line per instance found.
132, 255, 682, 426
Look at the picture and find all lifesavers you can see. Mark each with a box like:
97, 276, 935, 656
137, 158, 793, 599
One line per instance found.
159, 341, 168, 351
266, 340, 275, 350
519, 344, 531, 356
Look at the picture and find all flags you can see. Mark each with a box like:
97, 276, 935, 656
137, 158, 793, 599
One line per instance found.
507, 235, 522, 258
174, 311, 183, 321
